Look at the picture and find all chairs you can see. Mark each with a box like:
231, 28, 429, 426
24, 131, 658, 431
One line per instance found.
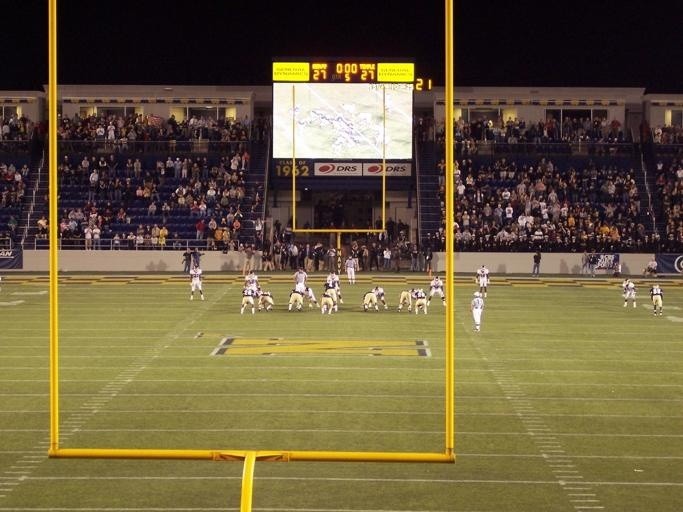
1, 116, 266, 257
411, 130, 681, 255
